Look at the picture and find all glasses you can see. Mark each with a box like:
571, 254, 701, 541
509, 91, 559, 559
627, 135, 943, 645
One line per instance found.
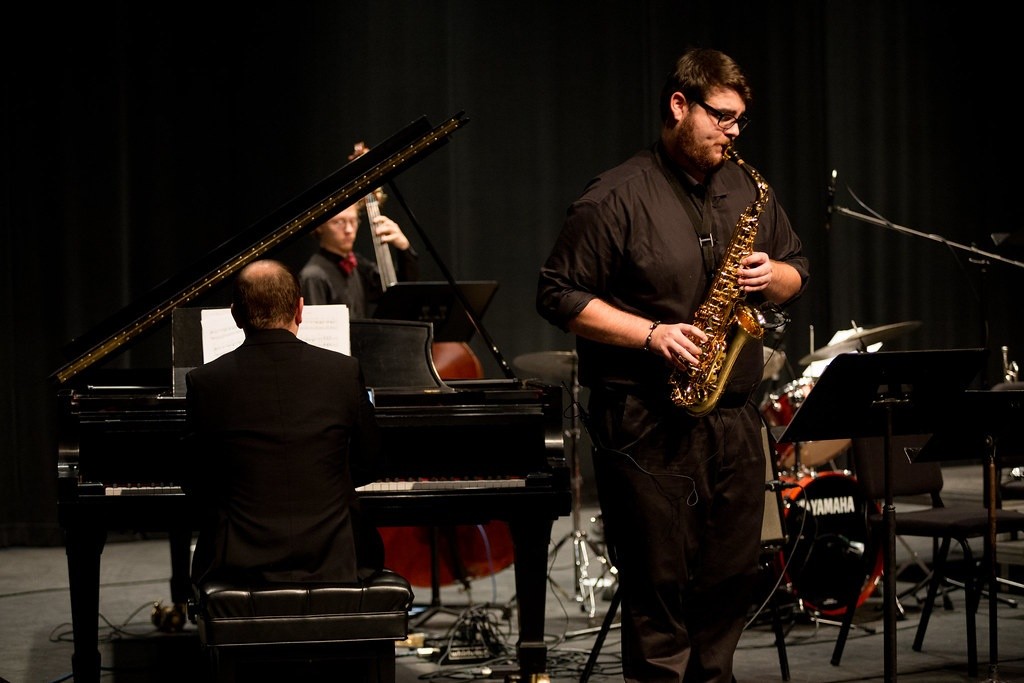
693, 97, 753, 130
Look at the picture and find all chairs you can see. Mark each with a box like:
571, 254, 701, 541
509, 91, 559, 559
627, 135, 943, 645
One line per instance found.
830, 434, 1024, 677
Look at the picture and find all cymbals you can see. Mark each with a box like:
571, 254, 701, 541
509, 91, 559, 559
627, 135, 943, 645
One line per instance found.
511, 345, 578, 382
796, 319, 923, 367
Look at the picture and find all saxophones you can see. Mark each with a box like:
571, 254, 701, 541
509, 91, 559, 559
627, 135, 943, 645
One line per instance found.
668, 143, 770, 417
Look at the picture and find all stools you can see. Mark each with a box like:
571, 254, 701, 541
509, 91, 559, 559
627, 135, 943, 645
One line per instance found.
200, 566, 414, 683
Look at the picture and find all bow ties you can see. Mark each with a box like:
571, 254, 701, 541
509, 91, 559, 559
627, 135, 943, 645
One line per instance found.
337, 251, 358, 277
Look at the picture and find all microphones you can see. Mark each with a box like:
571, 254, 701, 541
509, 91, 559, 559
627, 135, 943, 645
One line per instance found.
825, 173, 836, 220
760, 301, 777, 310
765, 480, 800, 491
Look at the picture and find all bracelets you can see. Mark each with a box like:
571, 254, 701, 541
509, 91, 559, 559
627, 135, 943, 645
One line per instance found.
643, 321, 660, 352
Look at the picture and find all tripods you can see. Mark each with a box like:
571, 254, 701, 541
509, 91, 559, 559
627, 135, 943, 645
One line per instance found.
547, 366, 619, 627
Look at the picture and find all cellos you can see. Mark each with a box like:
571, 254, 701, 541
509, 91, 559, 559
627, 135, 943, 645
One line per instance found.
347, 140, 514, 609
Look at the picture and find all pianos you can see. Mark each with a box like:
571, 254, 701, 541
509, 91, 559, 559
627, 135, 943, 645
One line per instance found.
43, 102, 577, 678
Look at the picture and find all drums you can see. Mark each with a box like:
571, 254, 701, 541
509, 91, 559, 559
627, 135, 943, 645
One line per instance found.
768, 465, 896, 618
764, 369, 852, 469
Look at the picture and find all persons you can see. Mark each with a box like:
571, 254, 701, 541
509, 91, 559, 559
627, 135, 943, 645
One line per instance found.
537, 50, 808, 683
183, 260, 385, 587
297, 199, 418, 338
